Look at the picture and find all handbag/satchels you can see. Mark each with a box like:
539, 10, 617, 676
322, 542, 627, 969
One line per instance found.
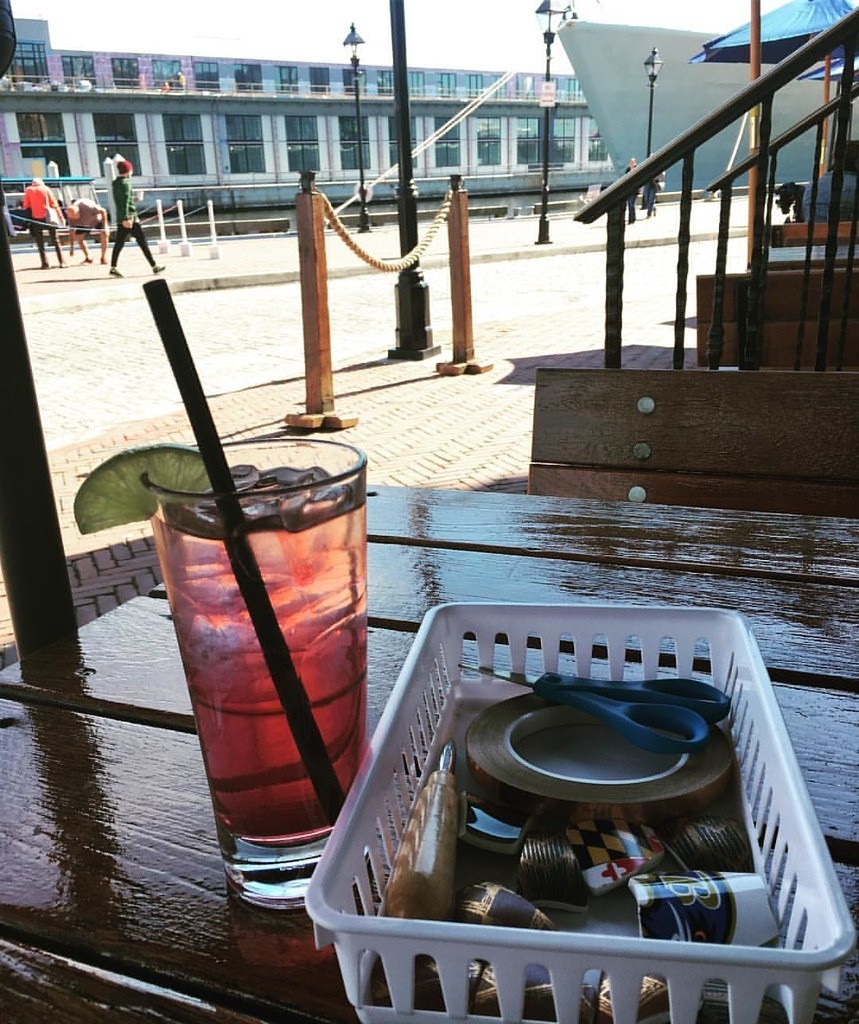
45, 186, 62, 226
655, 182, 665, 191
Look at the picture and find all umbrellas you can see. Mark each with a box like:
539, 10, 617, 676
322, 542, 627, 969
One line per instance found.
688, 0, 859, 64
795, 56, 859, 82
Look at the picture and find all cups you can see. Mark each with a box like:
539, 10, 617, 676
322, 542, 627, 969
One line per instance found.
140, 438, 368, 909
136, 190, 143, 201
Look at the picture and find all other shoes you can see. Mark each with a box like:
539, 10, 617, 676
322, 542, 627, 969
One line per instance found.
110, 269, 121, 276
43, 265, 51, 269
85, 258, 92, 263
153, 266, 165, 273
60, 264, 70, 267
101, 258, 108, 264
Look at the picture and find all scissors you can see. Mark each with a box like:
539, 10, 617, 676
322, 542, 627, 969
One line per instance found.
458, 662, 733, 753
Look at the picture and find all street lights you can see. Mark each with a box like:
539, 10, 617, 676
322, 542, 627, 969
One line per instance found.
534, 0, 566, 244
641, 47, 663, 210
343, 22, 371, 232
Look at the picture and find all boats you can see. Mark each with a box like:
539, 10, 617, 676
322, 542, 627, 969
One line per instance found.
0, 176, 131, 248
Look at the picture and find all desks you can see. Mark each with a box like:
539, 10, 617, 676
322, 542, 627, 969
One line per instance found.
0, 485, 859, 1024
748, 244, 858, 271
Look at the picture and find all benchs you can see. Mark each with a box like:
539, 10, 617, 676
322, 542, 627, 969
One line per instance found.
526, 366, 859, 521
696, 222, 859, 367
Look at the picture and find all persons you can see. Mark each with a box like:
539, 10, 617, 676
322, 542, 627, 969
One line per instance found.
23, 178, 66, 269
110, 161, 167, 277
160, 71, 186, 93
644, 153, 660, 219
67, 198, 110, 264
802, 141, 859, 222
625, 157, 641, 224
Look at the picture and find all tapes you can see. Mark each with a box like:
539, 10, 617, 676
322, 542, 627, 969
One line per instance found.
464, 691, 736, 822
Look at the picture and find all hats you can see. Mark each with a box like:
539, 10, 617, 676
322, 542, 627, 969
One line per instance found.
117, 162, 132, 174
